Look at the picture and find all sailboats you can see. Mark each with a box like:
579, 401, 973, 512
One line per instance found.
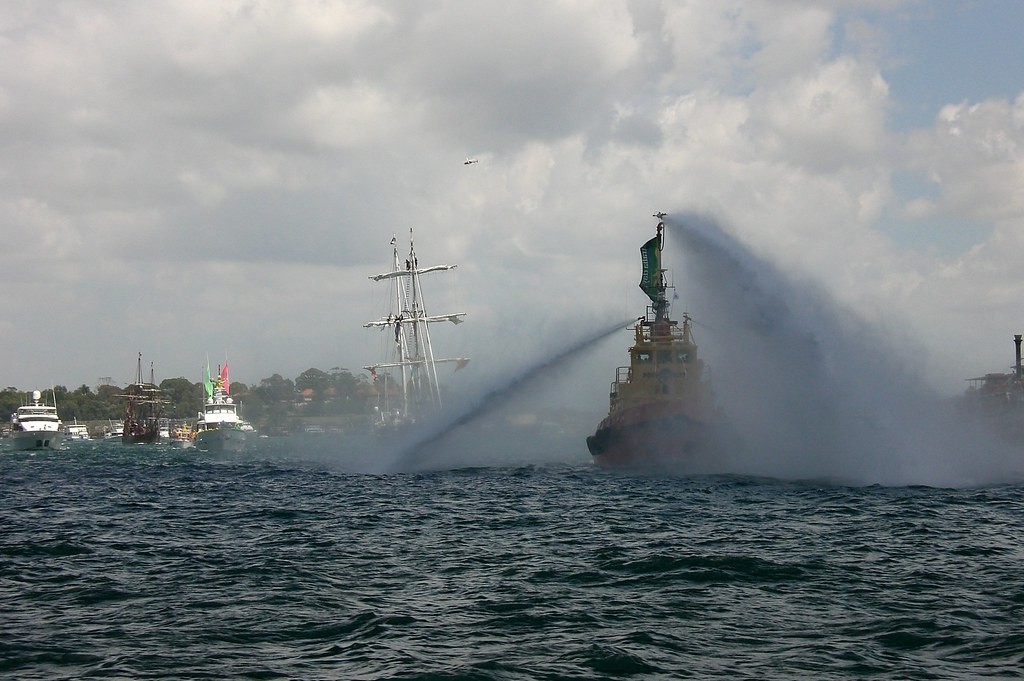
358, 228, 473, 472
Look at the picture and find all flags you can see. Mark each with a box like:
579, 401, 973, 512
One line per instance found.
204, 358, 213, 395
221, 360, 228, 393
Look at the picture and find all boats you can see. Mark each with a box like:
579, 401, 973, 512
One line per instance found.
941, 334, 1023, 484
585, 211, 756, 473
3, 352, 257, 456
